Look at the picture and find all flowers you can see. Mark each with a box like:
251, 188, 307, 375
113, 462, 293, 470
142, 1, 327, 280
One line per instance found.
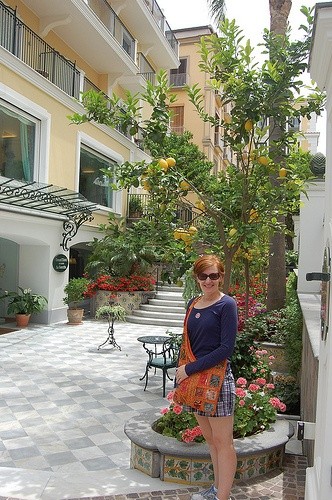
82, 273, 156, 300
157, 347, 300, 444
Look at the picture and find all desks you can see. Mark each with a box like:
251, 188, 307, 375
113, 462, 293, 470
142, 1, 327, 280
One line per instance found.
137, 336, 175, 381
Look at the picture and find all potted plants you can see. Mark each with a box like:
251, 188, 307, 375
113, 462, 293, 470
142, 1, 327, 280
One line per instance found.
129, 195, 143, 218
61, 277, 91, 324
94, 305, 127, 323
0, 286, 48, 328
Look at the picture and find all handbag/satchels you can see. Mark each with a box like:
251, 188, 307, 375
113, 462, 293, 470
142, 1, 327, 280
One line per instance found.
173, 331, 226, 415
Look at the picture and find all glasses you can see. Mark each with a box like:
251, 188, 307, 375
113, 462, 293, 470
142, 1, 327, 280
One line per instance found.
198, 272, 221, 281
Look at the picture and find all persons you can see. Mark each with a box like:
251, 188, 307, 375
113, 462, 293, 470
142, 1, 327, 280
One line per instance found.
174, 256, 238, 500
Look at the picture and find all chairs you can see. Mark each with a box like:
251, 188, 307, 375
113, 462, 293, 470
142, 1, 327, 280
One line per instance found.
144, 337, 181, 397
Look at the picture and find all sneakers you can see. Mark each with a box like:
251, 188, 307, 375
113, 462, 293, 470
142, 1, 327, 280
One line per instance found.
191, 482, 233, 500
